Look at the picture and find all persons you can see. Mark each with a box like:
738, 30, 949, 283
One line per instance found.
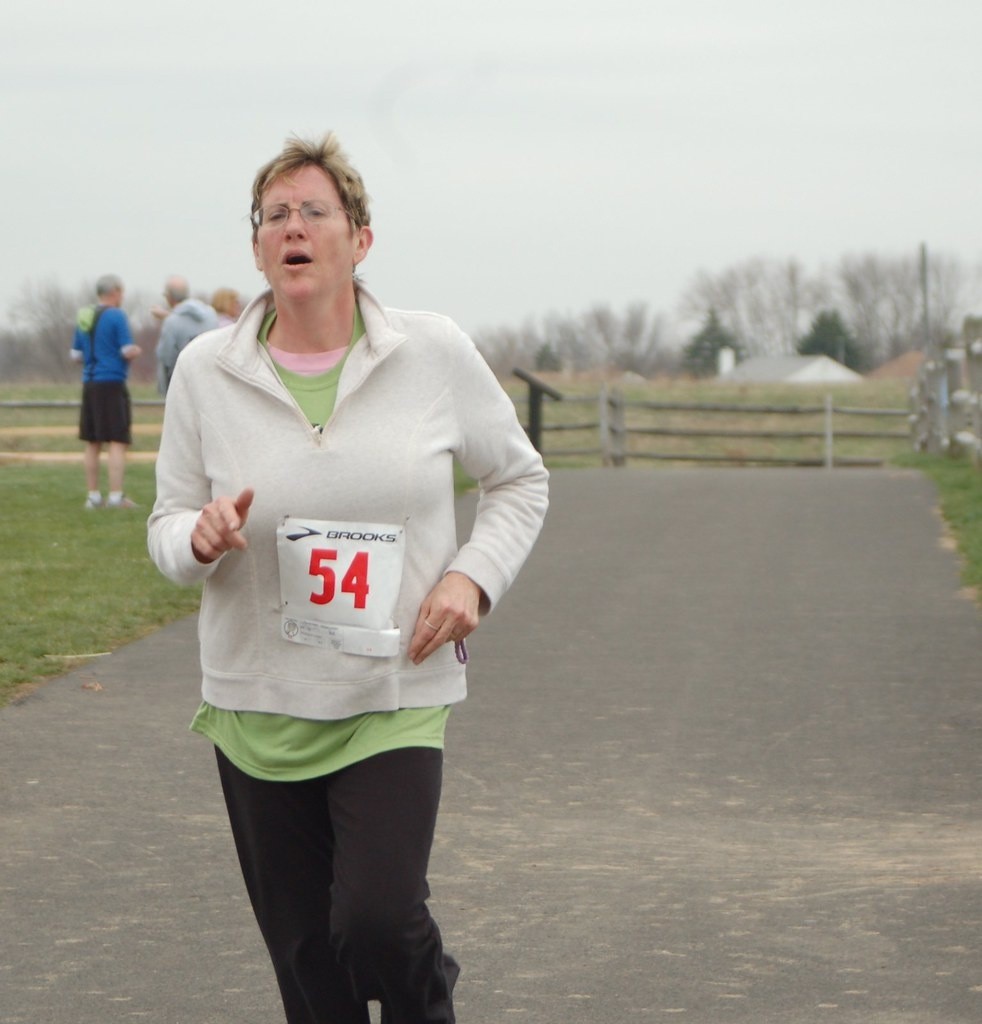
70, 274, 143, 511
151, 277, 242, 395
146, 128, 551, 1023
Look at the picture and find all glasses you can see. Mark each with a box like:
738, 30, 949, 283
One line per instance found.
249, 198, 359, 231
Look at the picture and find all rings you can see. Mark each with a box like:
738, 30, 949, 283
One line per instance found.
424, 620, 439, 632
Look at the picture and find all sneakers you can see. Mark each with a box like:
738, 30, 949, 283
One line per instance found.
84, 494, 141, 510
